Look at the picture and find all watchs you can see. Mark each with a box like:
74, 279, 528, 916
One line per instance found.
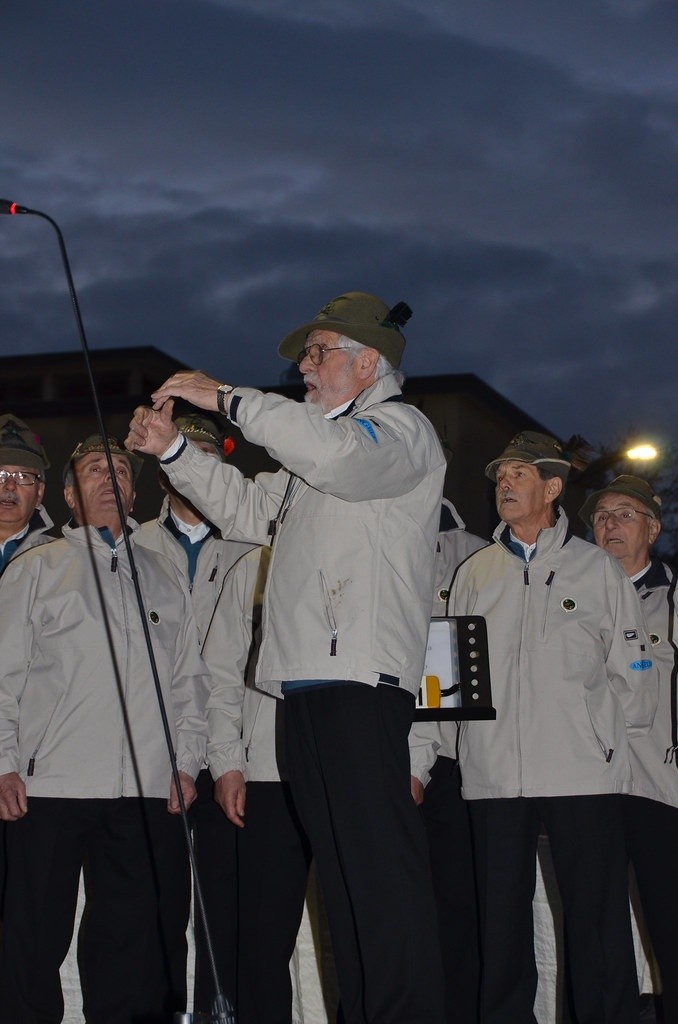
216, 384, 234, 415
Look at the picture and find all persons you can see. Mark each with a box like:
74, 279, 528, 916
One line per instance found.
124, 292, 447, 1024
0, 410, 678, 1024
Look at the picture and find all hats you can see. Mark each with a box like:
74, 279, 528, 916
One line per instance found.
175, 414, 226, 463
578, 474, 661, 531
0, 413, 51, 470
63, 432, 144, 484
485, 431, 572, 482
277, 291, 413, 370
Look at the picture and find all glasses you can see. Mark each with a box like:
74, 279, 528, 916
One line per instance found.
589, 507, 654, 526
297, 343, 357, 367
0, 469, 44, 485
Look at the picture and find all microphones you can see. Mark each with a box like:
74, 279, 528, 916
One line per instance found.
0, 199, 30, 215
